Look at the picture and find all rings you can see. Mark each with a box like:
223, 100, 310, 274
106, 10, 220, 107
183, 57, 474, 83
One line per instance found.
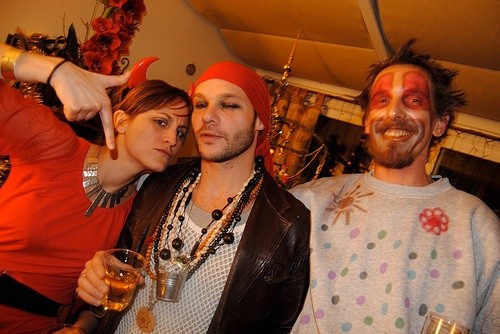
78, 270, 91, 279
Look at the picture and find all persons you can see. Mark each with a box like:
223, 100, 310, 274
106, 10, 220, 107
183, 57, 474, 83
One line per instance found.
278, 39, 499, 334
322, 147, 364, 175
0, 0, 193, 333
75, 61, 311, 334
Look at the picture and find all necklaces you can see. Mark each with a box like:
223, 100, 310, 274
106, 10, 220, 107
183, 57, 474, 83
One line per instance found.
128, 153, 267, 331
78, 141, 139, 219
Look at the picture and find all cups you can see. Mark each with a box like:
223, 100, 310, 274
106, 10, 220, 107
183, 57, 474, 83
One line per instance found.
420, 312, 470, 334
157, 271, 187, 302
101, 249, 147, 312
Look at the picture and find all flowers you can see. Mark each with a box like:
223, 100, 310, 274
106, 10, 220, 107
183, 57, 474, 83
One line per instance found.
79, 0, 147, 74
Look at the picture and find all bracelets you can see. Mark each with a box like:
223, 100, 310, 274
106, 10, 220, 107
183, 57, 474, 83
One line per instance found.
0, 41, 28, 77
40, 56, 75, 83
